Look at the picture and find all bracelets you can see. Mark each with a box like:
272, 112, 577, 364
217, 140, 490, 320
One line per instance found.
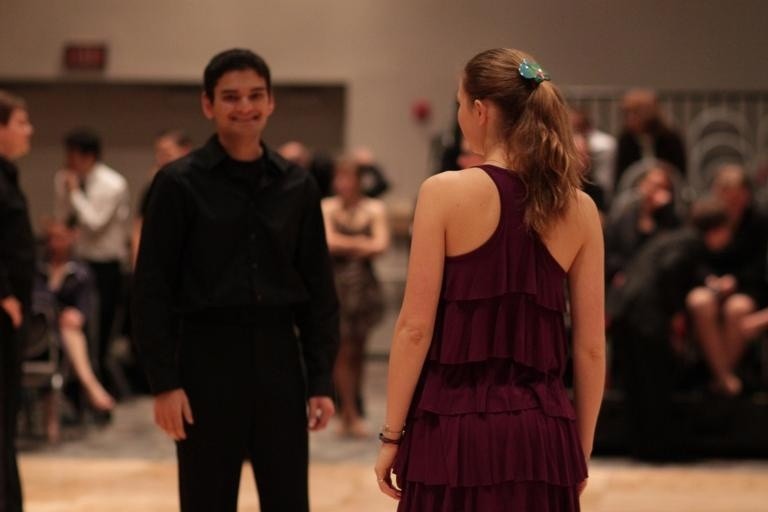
382, 426, 405, 434
379, 433, 400, 444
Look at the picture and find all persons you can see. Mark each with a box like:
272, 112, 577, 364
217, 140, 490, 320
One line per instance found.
130, 129, 199, 267
566, 104, 620, 204
571, 132, 606, 229
374, 47, 607, 512
607, 89, 767, 462
319, 162, 391, 440
0, 91, 36, 512
32, 222, 116, 446
277, 140, 314, 174
54, 126, 133, 426
129, 49, 337, 511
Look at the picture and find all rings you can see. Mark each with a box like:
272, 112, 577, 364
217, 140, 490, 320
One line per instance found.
379, 479, 384, 484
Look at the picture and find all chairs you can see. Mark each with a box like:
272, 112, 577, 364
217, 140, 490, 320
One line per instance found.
18, 291, 76, 447
